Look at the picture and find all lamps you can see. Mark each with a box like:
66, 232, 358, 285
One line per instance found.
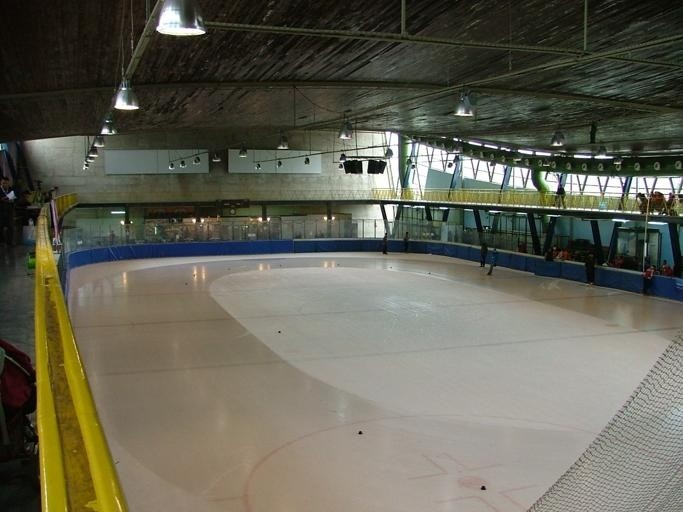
451, 86, 474, 117
153, 1, 206, 38
337, 122, 353, 140
276, 135, 289, 150
100, 78, 139, 136
238, 144, 247, 158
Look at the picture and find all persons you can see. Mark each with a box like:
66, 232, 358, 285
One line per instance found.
0, 177, 31, 250
404, 232, 410, 252
516, 184, 683, 298
382, 233, 387, 254
480, 243, 488, 268
